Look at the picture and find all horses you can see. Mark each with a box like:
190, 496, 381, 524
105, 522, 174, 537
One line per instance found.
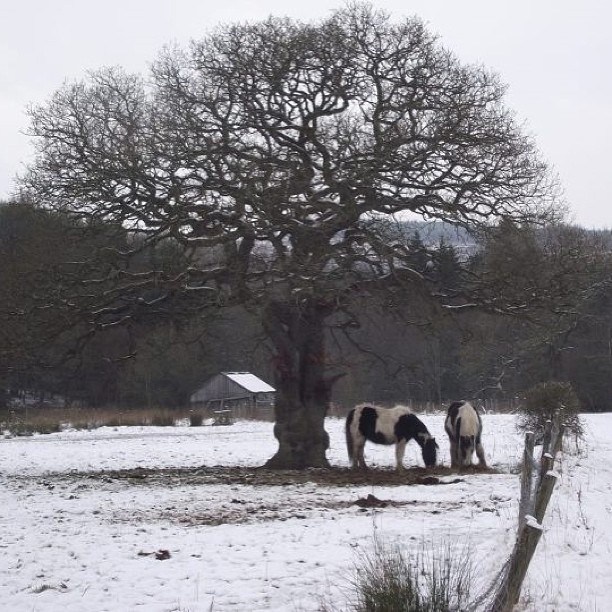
344, 402, 440, 469
444, 399, 487, 468
